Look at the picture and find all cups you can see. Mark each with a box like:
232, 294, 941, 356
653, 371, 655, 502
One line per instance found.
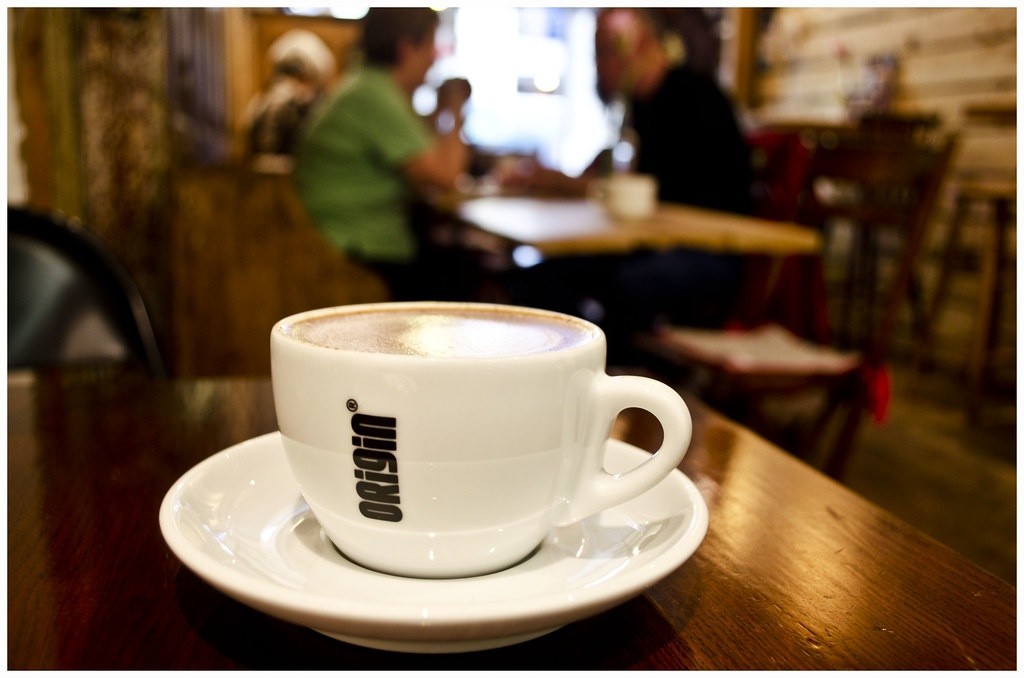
600, 174, 655, 224
271, 302, 692, 578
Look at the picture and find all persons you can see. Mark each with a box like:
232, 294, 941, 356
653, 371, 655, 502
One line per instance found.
499, 7, 757, 364
237, 8, 472, 304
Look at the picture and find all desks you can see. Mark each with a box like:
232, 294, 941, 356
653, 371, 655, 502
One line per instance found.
7, 368, 1016, 673
409, 193, 817, 381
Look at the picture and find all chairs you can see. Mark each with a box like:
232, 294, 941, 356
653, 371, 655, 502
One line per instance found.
7, 203, 158, 364
673, 124, 956, 473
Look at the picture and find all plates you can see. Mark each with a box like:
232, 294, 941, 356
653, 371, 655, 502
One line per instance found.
159, 430, 708, 654
